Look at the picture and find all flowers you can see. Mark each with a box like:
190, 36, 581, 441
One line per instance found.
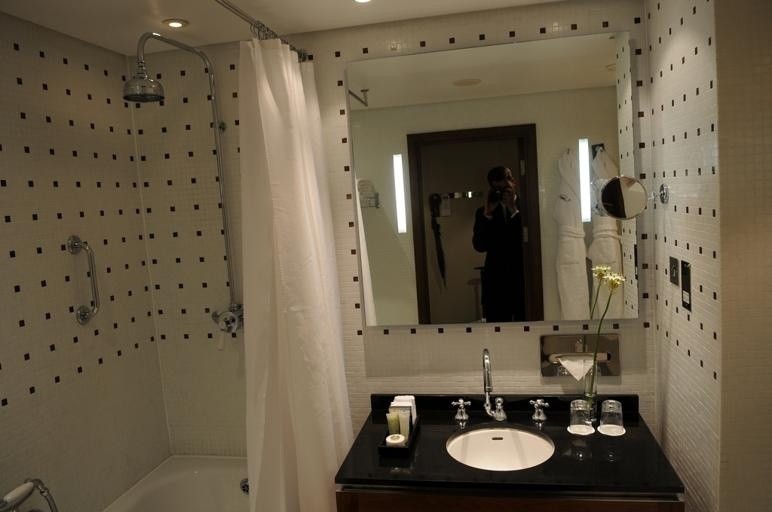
598, 273, 626, 339
590, 266, 610, 319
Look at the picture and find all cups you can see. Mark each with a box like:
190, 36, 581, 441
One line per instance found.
569, 398, 593, 434
598, 400, 624, 434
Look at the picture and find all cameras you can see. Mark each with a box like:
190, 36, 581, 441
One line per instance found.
488, 186, 509, 205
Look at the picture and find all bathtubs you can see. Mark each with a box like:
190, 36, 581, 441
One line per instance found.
103, 454, 248, 512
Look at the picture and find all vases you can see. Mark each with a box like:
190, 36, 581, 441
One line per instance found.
585, 362, 598, 414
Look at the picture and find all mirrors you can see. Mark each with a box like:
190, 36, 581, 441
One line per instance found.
344, 31, 640, 326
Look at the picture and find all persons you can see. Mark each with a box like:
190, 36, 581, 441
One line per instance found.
471, 164, 527, 322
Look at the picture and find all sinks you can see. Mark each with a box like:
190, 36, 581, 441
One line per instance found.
444, 422, 555, 473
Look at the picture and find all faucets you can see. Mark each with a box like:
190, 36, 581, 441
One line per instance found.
483, 348, 507, 422
